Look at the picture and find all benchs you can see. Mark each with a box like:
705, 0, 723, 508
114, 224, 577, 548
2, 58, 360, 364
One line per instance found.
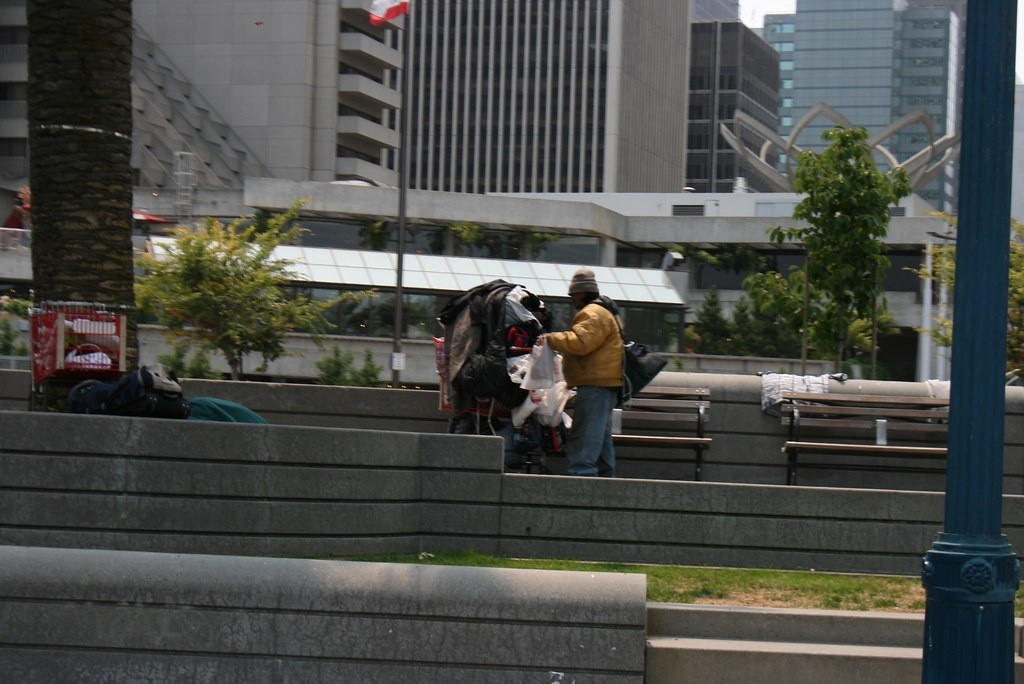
780, 391, 951, 486
515, 385, 714, 481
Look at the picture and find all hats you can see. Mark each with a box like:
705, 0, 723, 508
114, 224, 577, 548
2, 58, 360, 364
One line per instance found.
568, 267, 599, 295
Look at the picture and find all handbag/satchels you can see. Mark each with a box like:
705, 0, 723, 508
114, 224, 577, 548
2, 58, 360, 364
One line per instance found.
520, 335, 555, 390
622, 343, 668, 403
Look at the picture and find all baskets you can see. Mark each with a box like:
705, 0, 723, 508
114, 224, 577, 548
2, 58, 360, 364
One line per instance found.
29, 309, 127, 389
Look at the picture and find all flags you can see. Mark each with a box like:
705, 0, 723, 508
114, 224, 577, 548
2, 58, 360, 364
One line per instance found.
369, 0, 409, 27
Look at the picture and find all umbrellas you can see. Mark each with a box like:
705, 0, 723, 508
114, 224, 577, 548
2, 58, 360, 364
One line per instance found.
132, 209, 167, 224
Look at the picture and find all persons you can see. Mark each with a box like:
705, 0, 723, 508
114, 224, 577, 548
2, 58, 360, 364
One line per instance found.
536, 269, 624, 478
